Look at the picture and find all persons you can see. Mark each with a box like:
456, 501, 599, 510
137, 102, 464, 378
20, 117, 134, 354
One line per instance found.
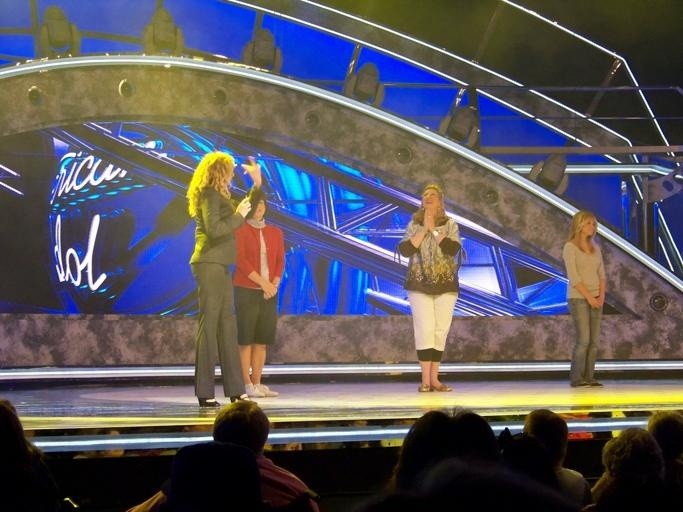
1, 396, 320, 510
397, 185, 463, 393
349, 406, 682, 511
186, 150, 263, 407
562, 208, 606, 388
229, 189, 285, 400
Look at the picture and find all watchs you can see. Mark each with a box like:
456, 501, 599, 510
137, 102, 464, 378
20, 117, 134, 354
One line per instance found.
431, 229, 439, 237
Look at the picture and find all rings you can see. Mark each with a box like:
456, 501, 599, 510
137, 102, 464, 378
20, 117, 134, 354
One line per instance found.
427, 215, 431, 218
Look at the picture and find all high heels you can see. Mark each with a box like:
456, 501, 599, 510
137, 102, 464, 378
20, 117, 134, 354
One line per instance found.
230, 395, 257, 405
418, 384, 453, 391
199, 398, 220, 407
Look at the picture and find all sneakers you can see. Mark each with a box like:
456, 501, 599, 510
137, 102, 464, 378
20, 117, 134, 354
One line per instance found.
245, 383, 279, 397
576, 381, 603, 387
